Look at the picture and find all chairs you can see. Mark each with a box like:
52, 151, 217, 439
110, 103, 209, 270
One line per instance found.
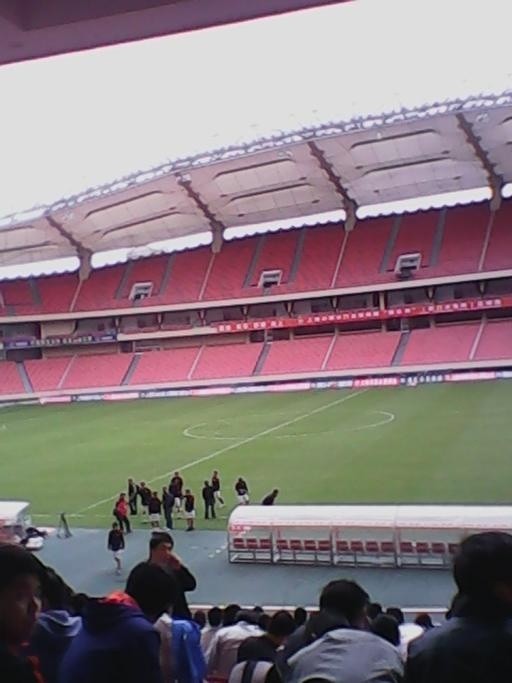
0, 193, 510, 396
228, 532, 466, 573
